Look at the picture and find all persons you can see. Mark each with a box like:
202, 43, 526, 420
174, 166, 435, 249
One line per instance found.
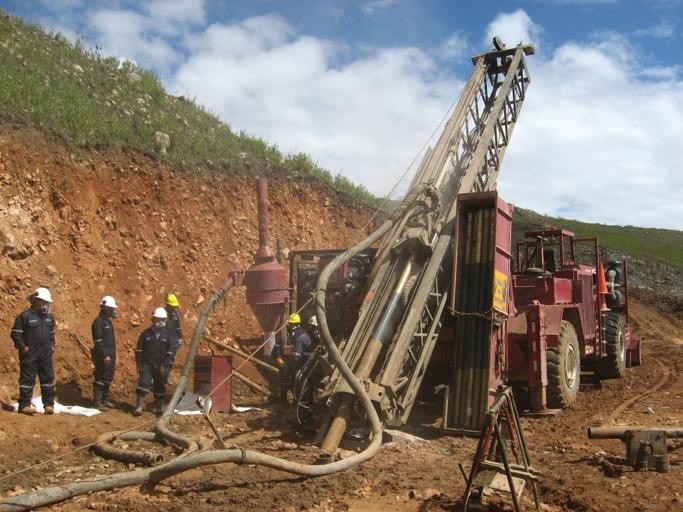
162, 292, 183, 387
306, 316, 323, 353
8, 285, 57, 415
269, 337, 299, 403
87, 293, 119, 413
286, 311, 311, 362
131, 307, 173, 417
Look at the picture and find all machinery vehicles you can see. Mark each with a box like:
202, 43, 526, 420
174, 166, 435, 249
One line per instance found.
217, 36, 642, 418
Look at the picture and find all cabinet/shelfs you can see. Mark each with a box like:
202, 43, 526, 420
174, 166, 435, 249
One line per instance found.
194, 355, 233, 414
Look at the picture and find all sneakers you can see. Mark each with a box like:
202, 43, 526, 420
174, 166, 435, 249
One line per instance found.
44, 406, 54, 414
18, 406, 36, 414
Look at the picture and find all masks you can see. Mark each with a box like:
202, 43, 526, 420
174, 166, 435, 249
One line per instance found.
155, 321, 166, 329
41, 304, 50, 314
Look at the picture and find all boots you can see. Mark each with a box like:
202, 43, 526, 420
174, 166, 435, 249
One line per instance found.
103, 385, 116, 408
91, 386, 110, 410
153, 398, 165, 414
133, 394, 145, 416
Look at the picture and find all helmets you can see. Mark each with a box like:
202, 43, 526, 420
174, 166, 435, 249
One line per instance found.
308, 315, 319, 327
166, 293, 180, 307
100, 296, 118, 308
152, 307, 168, 318
287, 313, 301, 324
34, 287, 54, 302
